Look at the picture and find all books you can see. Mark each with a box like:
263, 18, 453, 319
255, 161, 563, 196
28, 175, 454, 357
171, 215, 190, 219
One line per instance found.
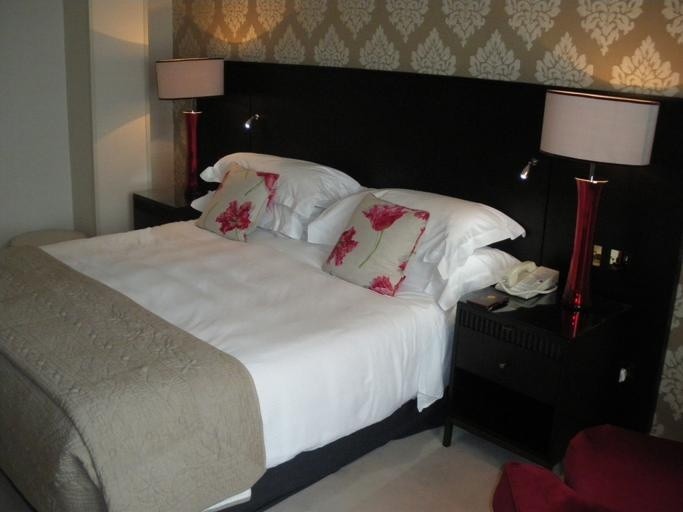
467, 291, 509, 312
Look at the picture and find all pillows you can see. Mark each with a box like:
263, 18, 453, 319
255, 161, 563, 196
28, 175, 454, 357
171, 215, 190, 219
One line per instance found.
402, 247, 523, 307
197, 160, 280, 242
191, 187, 323, 240
200, 153, 364, 218
308, 188, 529, 279
319, 191, 430, 297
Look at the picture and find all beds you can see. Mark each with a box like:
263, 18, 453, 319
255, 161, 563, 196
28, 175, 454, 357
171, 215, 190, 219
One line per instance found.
1, 217, 457, 512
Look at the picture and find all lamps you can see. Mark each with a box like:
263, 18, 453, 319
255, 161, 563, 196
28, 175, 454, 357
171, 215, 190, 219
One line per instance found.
536, 88, 660, 300
155, 57, 224, 204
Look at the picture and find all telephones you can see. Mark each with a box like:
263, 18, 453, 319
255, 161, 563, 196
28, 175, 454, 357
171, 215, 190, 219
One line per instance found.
495, 261, 559, 299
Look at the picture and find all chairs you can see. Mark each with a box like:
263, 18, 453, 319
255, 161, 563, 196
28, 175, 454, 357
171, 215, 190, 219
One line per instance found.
492, 423, 683, 512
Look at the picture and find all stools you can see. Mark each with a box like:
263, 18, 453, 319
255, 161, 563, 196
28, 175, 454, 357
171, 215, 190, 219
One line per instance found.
7, 228, 88, 248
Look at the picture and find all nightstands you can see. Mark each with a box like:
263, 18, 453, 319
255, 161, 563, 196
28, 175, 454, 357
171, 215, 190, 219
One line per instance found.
441, 271, 621, 471
132, 186, 214, 231
